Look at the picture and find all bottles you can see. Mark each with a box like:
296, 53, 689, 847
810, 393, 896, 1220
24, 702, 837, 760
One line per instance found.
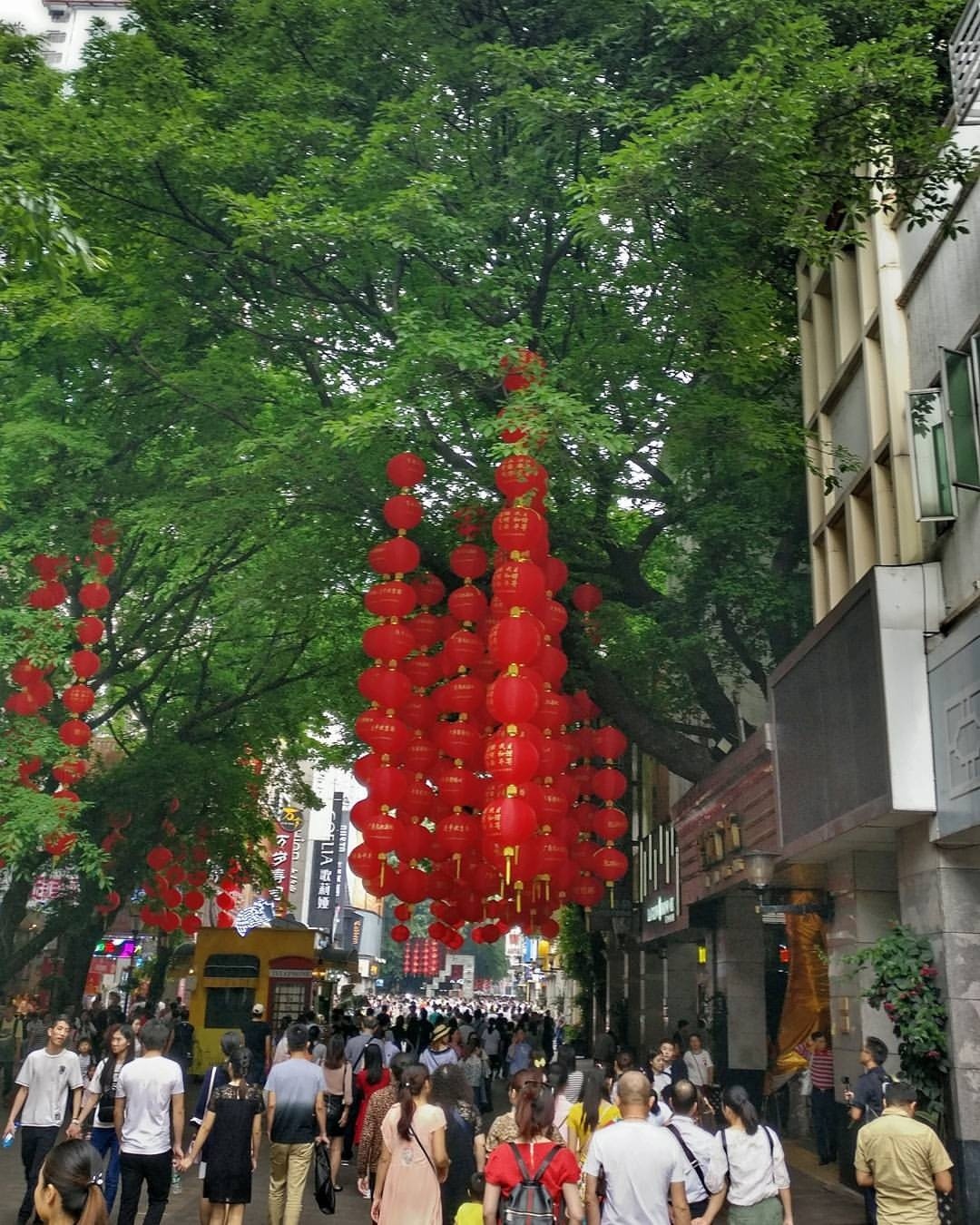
2, 1122, 19, 1147
172, 1158, 182, 1195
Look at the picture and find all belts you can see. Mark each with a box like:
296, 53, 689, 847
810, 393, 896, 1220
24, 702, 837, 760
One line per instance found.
812, 1088, 835, 1094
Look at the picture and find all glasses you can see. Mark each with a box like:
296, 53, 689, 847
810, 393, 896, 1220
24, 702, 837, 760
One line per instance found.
524, 1079, 555, 1095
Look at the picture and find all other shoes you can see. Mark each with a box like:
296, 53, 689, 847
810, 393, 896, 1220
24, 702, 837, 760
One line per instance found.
334, 1185, 343, 1193
340, 1160, 349, 1166
363, 1189, 372, 1199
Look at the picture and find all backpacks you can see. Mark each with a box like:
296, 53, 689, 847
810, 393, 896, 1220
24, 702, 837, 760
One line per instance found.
501, 1143, 566, 1225
98, 1059, 117, 1123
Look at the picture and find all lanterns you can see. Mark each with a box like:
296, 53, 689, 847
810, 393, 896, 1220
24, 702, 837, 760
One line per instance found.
0, 516, 241, 935
400, 937, 440, 976
346, 343, 628, 951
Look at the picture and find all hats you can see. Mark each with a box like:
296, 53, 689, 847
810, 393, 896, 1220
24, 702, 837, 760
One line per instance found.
252, 1003, 266, 1014
432, 1024, 451, 1041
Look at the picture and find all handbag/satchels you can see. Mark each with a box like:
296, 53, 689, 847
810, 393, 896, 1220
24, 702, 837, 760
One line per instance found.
701, 1082, 714, 1101
188, 1141, 202, 1165
799, 1052, 814, 1095
314, 1140, 336, 1215
182, 1052, 193, 1067
479, 1059, 494, 1113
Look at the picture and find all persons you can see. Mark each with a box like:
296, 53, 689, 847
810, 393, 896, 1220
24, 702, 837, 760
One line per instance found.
0, 990, 953, 1224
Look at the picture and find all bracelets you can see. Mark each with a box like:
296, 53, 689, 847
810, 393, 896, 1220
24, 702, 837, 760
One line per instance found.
71, 1117, 78, 1120
